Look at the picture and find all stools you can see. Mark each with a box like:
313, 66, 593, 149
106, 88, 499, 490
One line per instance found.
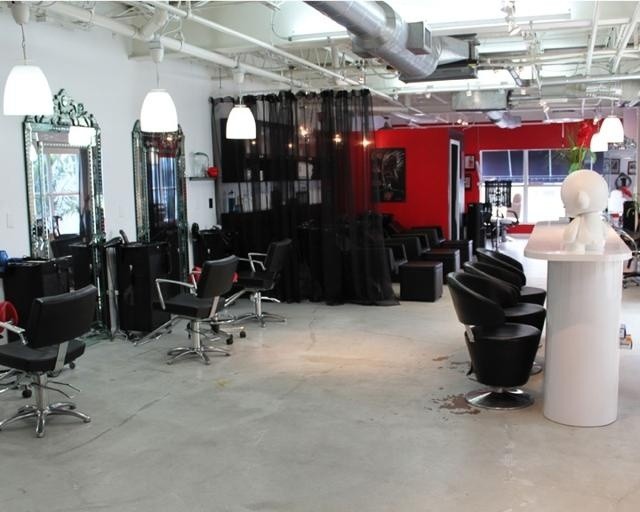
398, 240, 473, 302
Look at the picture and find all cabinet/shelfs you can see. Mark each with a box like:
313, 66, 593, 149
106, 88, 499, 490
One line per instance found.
0, 254, 74, 343
116, 242, 171, 332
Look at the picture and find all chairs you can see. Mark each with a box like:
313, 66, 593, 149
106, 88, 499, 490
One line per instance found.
151, 238, 292, 365
447, 248, 547, 411
0, 285, 98, 437
490, 193, 522, 243
300, 212, 446, 296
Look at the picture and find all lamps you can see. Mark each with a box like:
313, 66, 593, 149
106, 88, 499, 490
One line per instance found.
225, 70, 258, 140
590, 96, 624, 152
139, 44, 179, 133
2, 3, 54, 117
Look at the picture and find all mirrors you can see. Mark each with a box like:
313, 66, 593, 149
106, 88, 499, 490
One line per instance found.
133, 120, 190, 293
22, 89, 112, 346
